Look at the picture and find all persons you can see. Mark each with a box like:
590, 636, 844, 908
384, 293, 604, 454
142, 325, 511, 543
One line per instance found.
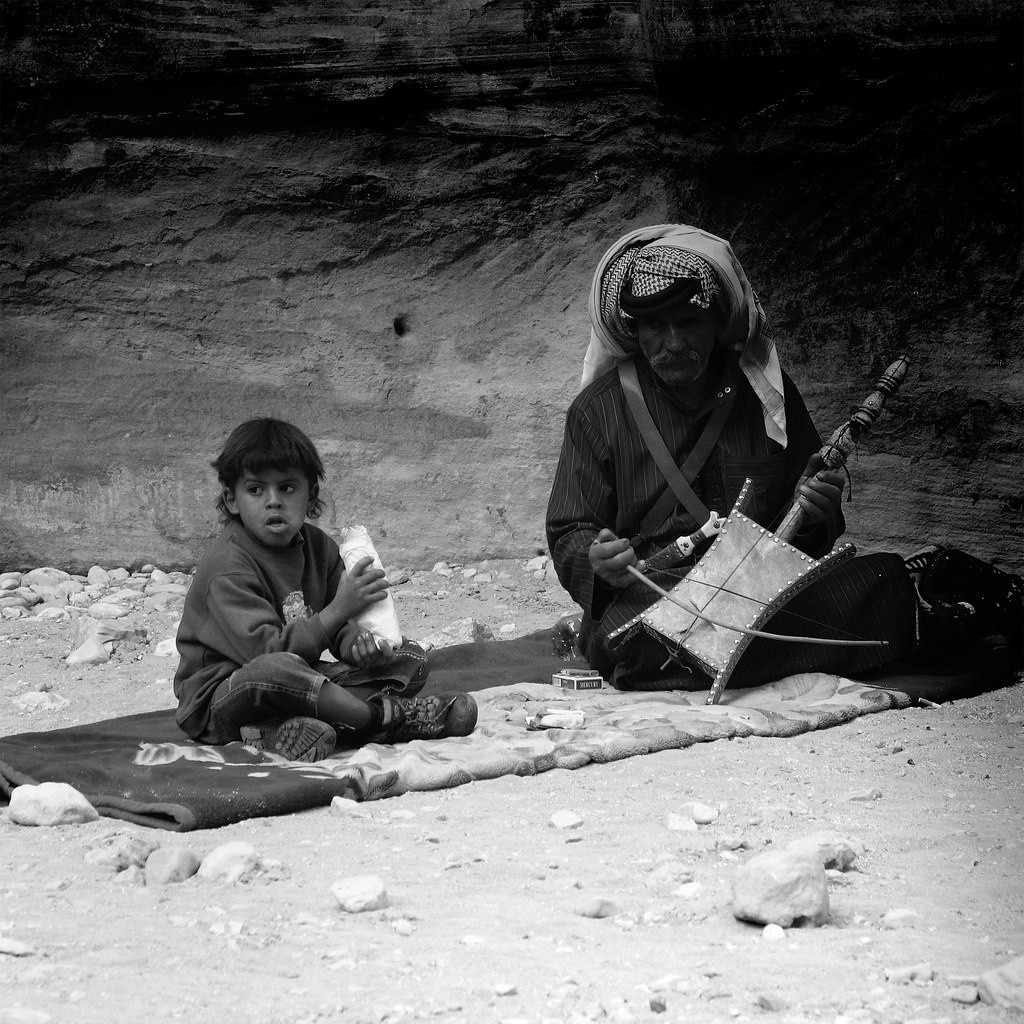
547, 223, 922, 692
174, 417, 478, 759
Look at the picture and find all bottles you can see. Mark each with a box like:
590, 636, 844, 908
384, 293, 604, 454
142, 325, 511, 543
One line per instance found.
551, 613, 584, 657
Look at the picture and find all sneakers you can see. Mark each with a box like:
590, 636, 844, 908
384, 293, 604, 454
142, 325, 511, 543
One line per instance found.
239, 715, 337, 763
365, 691, 478, 745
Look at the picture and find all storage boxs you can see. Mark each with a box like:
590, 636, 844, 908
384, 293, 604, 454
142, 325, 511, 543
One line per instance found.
551, 674, 603, 693
